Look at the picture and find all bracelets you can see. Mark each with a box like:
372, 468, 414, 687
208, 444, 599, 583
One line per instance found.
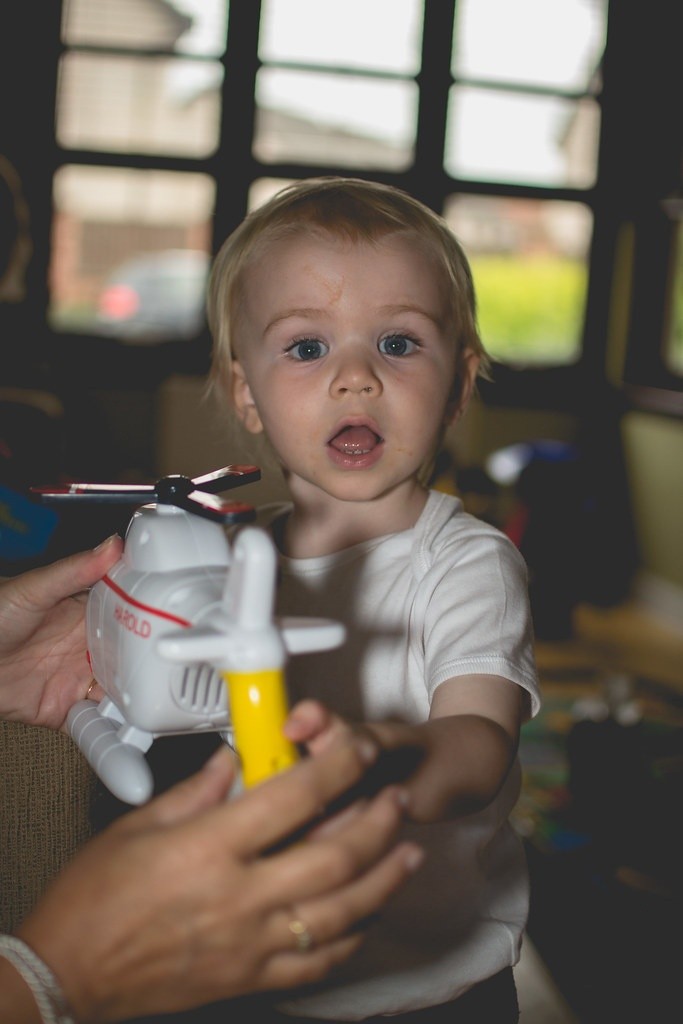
0, 931, 75, 1024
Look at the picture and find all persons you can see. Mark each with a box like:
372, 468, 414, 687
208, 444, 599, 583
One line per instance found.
167, 176, 543, 1024
0, 534, 424, 1024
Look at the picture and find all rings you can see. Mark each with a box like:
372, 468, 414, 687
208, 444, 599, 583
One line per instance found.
85, 677, 98, 702
276, 905, 312, 954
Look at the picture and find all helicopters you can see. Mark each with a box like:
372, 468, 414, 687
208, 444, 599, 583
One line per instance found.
29, 464, 347, 813
